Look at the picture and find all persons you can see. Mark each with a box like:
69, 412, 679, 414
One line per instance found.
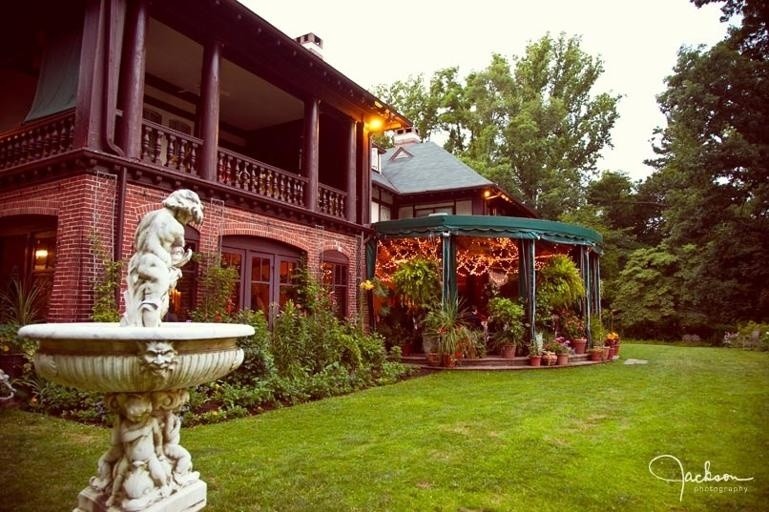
119, 188, 204, 326
87, 395, 193, 507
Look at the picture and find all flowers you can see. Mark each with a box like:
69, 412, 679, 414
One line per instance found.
567, 316, 586, 338
606, 332, 620, 346
555, 335, 575, 353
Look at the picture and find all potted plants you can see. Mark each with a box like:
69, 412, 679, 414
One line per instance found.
1, 277, 51, 385
528, 327, 559, 366
391, 253, 526, 367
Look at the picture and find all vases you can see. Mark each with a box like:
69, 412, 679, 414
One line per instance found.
557, 353, 569, 364
591, 345, 620, 362
575, 339, 587, 354
